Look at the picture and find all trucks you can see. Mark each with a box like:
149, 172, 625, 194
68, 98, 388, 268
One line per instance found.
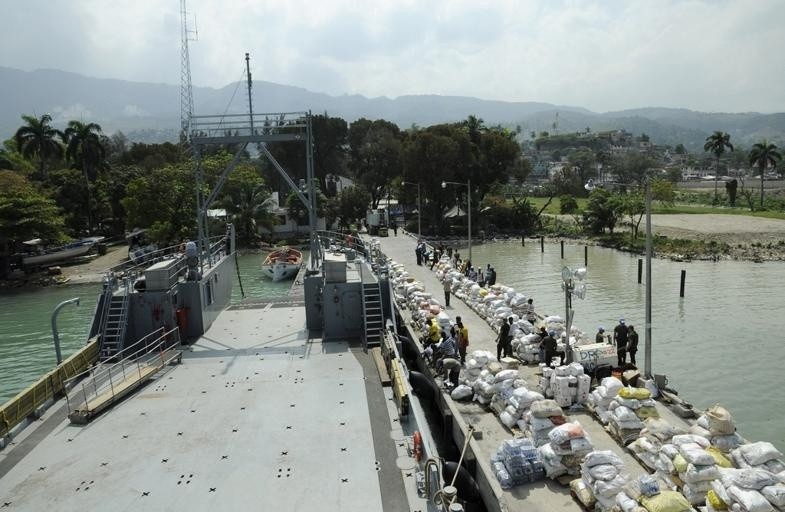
366, 209, 389, 235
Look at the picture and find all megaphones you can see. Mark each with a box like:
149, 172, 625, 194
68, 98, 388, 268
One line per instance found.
568, 285, 586, 300
571, 267, 587, 281
561, 266, 574, 282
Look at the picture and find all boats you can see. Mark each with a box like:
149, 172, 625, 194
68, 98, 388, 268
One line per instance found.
261, 247, 303, 281
22, 231, 106, 269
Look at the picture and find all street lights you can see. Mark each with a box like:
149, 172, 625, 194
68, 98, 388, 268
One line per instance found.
441, 179, 471, 266
584, 177, 651, 380
401, 181, 421, 241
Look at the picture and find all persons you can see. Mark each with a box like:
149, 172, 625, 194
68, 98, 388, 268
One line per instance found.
524, 299, 535, 325
441, 274, 453, 306
495, 317, 529, 361
419, 317, 469, 387
394, 224, 397, 236
596, 318, 638, 366
415, 238, 496, 287
179, 237, 196, 251
536, 327, 565, 369
